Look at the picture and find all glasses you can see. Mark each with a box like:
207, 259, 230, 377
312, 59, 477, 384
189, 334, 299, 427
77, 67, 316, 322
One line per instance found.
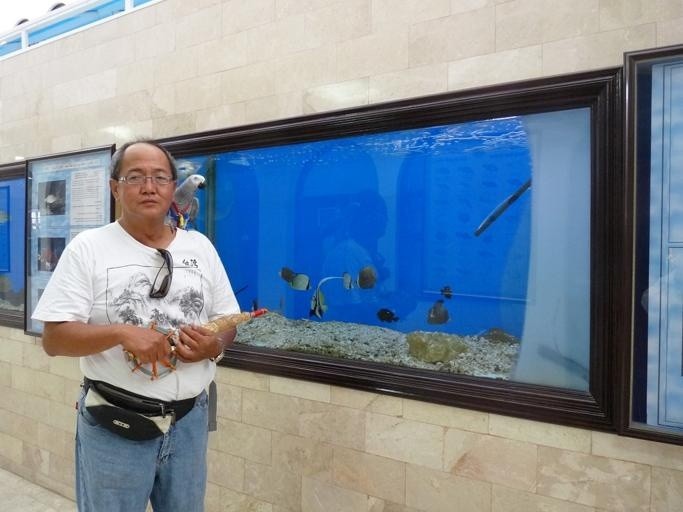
150, 248, 173, 297
117, 176, 173, 184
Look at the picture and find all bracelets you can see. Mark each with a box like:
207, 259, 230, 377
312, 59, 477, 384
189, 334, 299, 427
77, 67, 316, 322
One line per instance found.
208, 350, 224, 364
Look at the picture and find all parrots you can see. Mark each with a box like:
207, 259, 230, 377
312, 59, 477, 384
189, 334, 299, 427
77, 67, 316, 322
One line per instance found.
166, 174, 208, 234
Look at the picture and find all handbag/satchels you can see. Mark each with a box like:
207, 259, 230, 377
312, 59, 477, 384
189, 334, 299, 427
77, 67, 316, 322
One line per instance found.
79, 377, 195, 442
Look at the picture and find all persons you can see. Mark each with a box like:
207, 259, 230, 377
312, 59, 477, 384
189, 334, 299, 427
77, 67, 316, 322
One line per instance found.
27, 132, 241, 512
